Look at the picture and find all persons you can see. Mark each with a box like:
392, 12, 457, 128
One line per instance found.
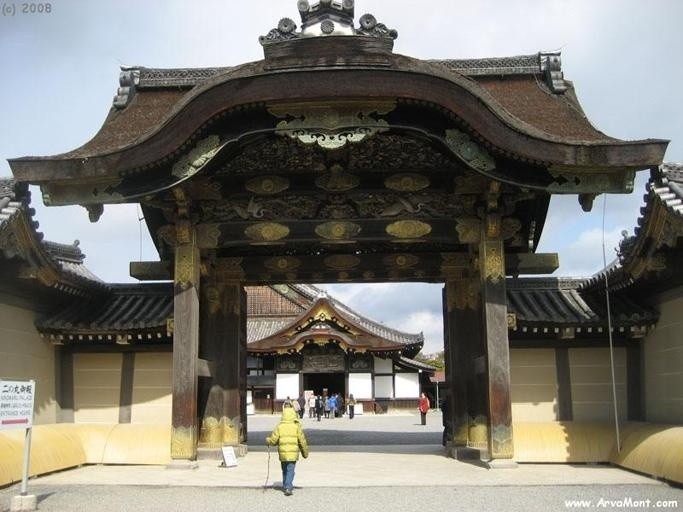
314, 394, 323, 421
265, 406, 308, 495
418, 392, 430, 425
297, 391, 306, 417
322, 392, 344, 419
307, 393, 316, 418
346, 393, 356, 419
283, 395, 291, 406
290, 399, 300, 414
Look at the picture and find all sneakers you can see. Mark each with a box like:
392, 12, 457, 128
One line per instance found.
281, 486, 293, 496
